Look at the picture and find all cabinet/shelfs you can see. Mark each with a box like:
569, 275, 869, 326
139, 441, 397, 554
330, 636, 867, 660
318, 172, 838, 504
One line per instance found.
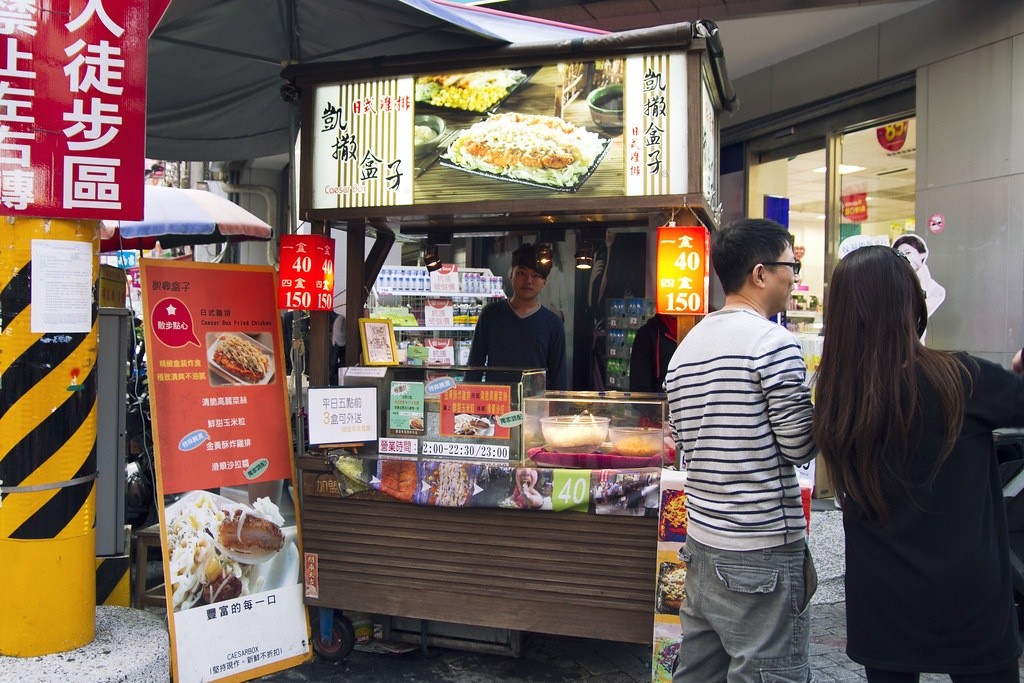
370, 266, 509, 367
606, 297, 656, 391
786, 289, 821, 325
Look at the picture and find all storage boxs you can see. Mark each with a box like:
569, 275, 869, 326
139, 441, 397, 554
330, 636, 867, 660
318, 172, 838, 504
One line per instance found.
453, 315, 470, 323
521, 389, 680, 470
470, 316, 479, 324
454, 340, 488, 366
406, 358, 422, 366
407, 345, 430, 358
387, 367, 547, 464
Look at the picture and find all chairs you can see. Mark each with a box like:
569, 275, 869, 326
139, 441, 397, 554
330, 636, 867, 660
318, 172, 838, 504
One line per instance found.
134, 523, 167, 610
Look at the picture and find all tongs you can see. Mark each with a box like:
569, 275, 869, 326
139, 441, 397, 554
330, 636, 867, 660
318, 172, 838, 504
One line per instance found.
571, 408, 598, 429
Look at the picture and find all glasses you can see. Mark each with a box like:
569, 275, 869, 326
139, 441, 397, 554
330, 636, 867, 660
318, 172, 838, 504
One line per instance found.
749, 258, 804, 273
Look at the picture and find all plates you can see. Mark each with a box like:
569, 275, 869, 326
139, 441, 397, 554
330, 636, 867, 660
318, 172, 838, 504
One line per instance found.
415, 65, 543, 115
207, 332, 274, 385
657, 561, 681, 615
438, 118, 612, 193
660, 488, 689, 545
165, 489, 303, 615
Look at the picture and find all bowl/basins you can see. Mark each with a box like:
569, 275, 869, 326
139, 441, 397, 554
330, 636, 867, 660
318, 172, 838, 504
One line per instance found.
540, 415, 610, 454
587, 84, 623, 134
524, 415, 539, 445
415, 114, 445, 160
608, 427, 661, 457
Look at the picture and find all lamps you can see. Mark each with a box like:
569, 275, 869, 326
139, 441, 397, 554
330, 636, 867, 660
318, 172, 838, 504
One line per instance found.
574, 226, 608, 270
535, 229, 568, 267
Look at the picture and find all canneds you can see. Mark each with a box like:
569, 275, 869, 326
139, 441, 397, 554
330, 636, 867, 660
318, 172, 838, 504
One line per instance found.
380, 269, 503, 293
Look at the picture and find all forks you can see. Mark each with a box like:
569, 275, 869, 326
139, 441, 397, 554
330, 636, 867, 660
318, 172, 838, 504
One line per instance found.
207, 524, 298, 565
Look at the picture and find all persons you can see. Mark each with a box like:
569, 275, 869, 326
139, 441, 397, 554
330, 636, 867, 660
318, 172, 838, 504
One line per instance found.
630, 306, 716, 427
661, 219, 819, 683
891, 234, 946, 346
596, 476, 660, 517
814, 245, 1024, 683
464, 243, 568, 418
497, 468, 543, 508
281, 310, 346, 386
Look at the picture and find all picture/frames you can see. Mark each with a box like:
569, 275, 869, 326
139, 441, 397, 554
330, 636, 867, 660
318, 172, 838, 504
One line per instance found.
358, 317, 400, 366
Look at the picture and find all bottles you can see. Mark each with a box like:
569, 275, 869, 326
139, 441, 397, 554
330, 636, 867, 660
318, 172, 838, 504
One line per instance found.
607, 299, 656, 388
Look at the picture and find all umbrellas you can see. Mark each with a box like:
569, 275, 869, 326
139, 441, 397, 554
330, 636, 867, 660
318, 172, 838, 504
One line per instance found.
100, 185, 273, 259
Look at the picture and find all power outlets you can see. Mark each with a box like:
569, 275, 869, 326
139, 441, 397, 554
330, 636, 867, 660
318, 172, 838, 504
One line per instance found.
423, 232, 454, 273
